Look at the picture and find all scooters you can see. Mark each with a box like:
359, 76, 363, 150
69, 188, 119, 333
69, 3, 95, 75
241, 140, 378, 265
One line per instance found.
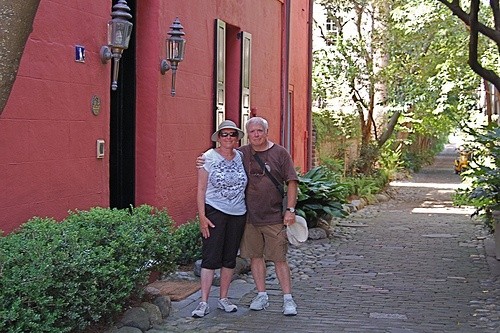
453, 150, 473, 176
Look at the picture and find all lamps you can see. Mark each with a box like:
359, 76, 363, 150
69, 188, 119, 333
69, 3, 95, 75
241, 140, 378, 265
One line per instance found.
100, 0, 133, 90
161, 17, 186, 97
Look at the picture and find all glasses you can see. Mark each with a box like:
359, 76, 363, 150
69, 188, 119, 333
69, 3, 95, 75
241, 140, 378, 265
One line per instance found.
218, 132, 239, 137
249, 161, 266, 177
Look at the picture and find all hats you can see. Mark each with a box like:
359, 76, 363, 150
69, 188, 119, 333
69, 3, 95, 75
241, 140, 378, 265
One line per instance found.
211, 120, 244, 141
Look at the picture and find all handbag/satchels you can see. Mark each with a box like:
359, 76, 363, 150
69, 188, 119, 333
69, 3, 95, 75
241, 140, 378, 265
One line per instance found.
286, 214, 309, 246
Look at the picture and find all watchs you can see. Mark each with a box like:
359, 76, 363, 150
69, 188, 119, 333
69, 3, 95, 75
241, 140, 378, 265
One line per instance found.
286, 208, 296, 213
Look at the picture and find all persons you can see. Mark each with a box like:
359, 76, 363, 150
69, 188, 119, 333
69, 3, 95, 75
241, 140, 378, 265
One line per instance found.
191, 120, 248, 317
195, 117, 299, 315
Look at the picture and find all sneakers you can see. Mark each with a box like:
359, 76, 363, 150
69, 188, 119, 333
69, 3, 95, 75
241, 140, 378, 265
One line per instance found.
249, 295, 269, 310
217, 298, 237, 313
281, 299, 298, 316
191, 302, 210, 318
